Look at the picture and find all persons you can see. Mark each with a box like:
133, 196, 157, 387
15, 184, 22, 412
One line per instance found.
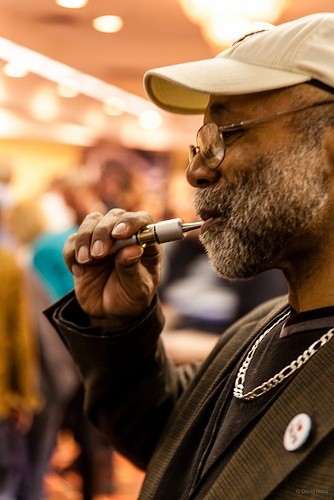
39, 11, 334, 500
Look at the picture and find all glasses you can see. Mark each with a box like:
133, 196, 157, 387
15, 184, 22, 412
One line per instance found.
187, 95, 333, 170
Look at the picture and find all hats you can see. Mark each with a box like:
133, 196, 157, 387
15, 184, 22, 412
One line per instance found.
143, 13, 334, 115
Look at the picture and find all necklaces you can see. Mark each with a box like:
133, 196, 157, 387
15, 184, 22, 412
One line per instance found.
230, 305, 334, 404
